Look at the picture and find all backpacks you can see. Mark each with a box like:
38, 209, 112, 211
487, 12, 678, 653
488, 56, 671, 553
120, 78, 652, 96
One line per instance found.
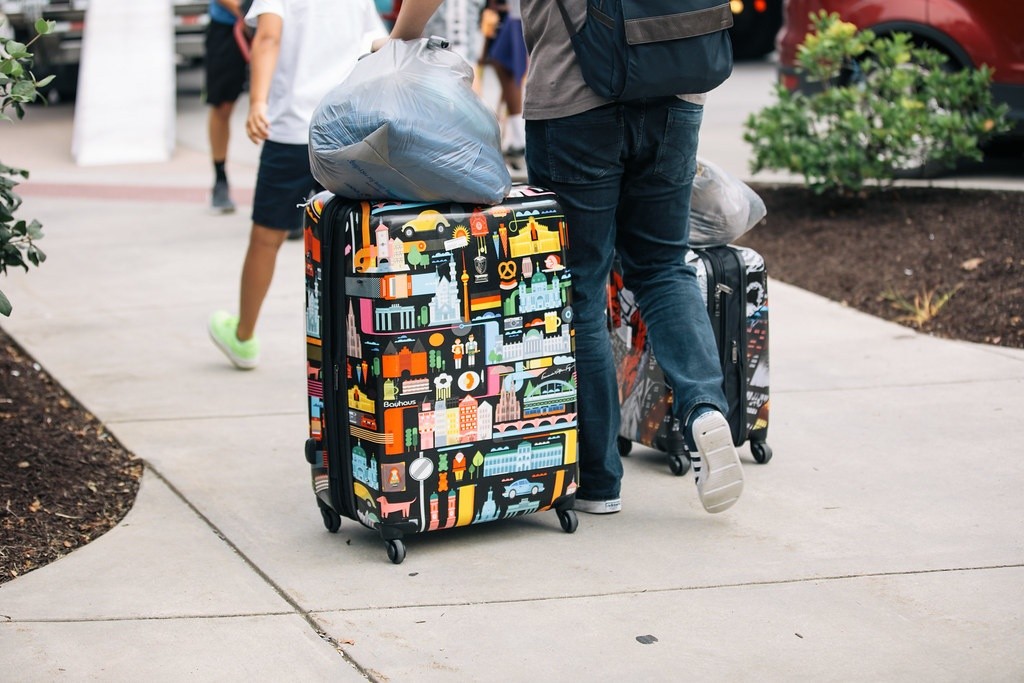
555, 0, 733, 103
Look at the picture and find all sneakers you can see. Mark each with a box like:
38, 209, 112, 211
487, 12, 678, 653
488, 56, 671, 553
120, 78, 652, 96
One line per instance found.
552, 492, 621, 514
207, 311, 257, 370
683, 406, 745, 513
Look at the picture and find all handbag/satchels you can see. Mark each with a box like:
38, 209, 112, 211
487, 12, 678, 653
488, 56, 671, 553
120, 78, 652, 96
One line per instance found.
309, 38, 513, 206
689, 159, 768, 246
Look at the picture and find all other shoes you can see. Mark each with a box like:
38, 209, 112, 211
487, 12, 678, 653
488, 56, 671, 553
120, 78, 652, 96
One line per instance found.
212, 181, 234, 213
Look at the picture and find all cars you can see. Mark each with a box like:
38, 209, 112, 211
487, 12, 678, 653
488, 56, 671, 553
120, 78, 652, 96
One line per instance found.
0, 0, 214, 106
768, 1, 1024, 183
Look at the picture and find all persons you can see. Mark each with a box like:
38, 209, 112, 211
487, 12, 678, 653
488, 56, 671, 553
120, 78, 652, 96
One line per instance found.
371, 0, 745, 515
200, 0, 530, 370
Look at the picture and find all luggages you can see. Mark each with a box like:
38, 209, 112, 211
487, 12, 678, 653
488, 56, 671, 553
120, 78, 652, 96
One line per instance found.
606, 245, 772, 477
299, 35, 578, 566
